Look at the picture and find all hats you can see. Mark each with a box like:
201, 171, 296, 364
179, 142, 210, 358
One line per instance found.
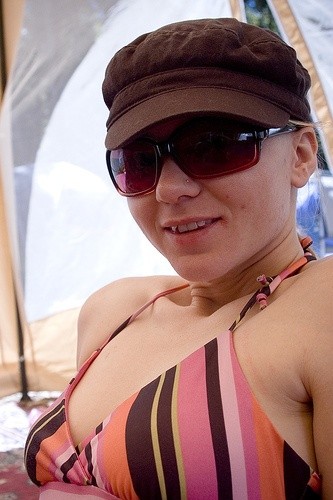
102, 17, 314, 152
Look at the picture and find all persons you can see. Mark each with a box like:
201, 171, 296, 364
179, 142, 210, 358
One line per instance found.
19, 16, 332, 499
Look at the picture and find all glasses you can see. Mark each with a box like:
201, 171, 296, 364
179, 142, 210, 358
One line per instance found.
105, 114, 300, 197
